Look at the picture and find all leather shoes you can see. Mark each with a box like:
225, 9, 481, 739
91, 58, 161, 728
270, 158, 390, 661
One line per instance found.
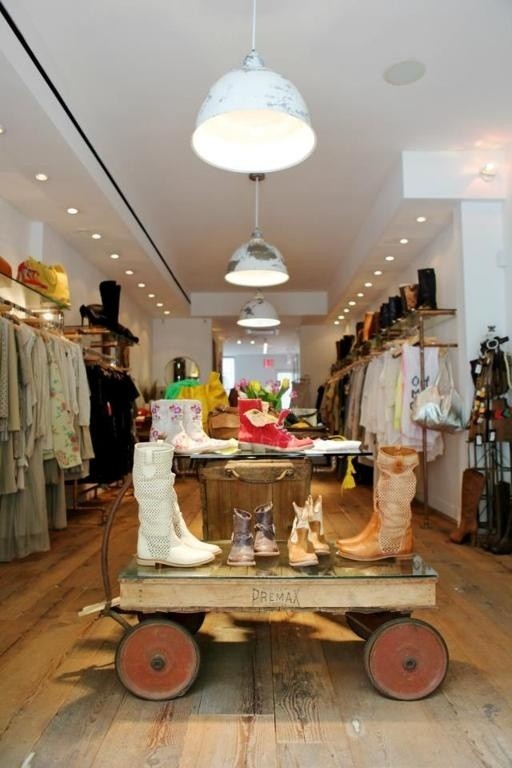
124, 328, 139, 343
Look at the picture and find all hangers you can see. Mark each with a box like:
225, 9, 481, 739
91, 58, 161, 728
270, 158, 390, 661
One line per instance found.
328, 324, 447, 383
0, 297, 132, 378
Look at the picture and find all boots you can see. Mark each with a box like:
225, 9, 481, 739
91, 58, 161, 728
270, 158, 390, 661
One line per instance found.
225, 507, 257, 568
483, 482, 512, 555
356, 285, 419, 348
415, 269, 436, 310
286, 495, 331, 567
100, 280, 126, 335
336, 335, 354, 361
238, 397, 314, 452
135, 438, 221, 568
449, 468, 485, 546
254, 503, 281, 558
150, 398, 236, 454
334, 445, 419, 561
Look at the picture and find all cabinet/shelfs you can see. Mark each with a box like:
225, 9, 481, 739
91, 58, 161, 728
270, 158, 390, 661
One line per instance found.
116, 439, 449, 702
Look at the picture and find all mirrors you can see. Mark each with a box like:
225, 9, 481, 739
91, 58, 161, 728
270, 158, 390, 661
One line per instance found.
164, 356, 200, 385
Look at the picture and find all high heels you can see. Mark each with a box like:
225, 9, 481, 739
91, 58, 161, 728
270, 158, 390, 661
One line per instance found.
79, 305, 108, 327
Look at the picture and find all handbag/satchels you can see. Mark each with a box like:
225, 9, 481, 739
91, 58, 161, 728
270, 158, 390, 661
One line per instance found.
471, 351, 511, 444
410, 386, 463, 433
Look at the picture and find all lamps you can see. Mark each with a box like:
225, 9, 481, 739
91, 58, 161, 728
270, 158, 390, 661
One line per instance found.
191, 1, 317, 177
220, 172, 292, 291
236, 286, 282, 328
